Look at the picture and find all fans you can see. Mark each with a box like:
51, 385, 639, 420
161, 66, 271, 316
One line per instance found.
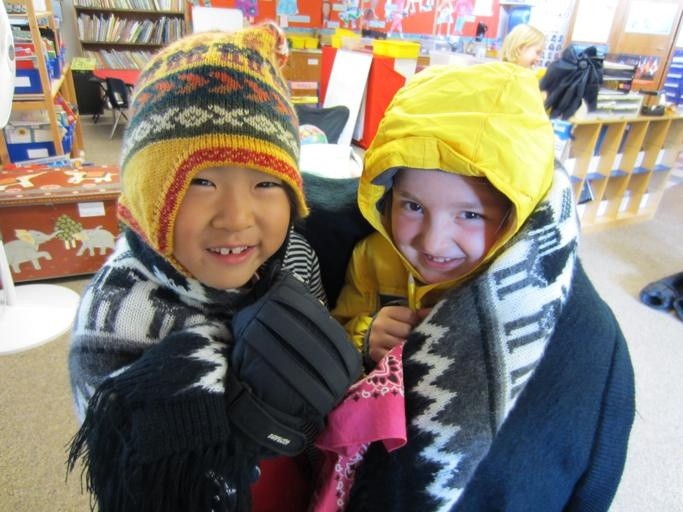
0, 0, 83, 361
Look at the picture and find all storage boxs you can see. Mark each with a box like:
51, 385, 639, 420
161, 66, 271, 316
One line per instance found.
278, 25, 422, 59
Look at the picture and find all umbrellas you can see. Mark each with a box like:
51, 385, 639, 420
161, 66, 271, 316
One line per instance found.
537, 43, 606, 122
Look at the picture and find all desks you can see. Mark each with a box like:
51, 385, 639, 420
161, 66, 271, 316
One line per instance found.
1, 162, 125, 290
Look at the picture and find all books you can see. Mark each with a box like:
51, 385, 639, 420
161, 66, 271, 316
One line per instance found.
3, 0, 186, 144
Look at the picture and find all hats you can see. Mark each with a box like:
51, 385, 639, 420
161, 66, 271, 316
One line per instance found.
115, 18, 310, 259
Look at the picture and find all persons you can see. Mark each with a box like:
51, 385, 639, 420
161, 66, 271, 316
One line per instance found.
501, 21, 547, 68
332, 57, 634, 511
71, 22, 330, 512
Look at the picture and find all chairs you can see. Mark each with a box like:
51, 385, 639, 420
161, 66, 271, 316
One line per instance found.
105, 76, 131, 139
291, 102, 352, 146
88, 75, 134, 124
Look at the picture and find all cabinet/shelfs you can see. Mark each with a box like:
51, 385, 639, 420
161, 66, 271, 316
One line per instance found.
0, 0, 89, 166
321, 45, 428, 151
277, 50, 319, 99
569, 114, 682, 231
71, 0, 192, 84
